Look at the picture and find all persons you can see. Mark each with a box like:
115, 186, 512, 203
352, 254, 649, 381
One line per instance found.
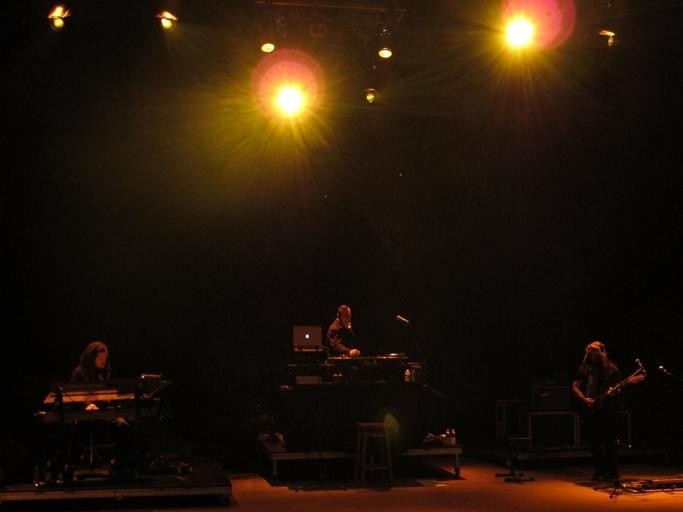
68, 341, 127, 466
571, 340, 623, 481
327, 304, 361, 356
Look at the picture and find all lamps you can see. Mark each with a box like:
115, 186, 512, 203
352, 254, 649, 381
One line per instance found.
373, 22, 396, 59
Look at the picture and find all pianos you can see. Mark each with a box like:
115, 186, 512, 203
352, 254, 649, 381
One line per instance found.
42, 382, 155, 423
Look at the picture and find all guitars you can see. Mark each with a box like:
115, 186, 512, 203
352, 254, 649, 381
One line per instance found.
583, 374, 646, 413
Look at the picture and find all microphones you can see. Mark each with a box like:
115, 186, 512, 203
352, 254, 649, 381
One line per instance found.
396, 315, 409, 326
659, 366, 670, 376
634, 356, 645, 372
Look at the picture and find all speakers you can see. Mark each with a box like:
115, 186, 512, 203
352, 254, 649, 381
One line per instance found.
287, 427, 330, 453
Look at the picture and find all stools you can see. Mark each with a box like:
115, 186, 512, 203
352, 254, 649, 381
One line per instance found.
352, 422, 393, 489
